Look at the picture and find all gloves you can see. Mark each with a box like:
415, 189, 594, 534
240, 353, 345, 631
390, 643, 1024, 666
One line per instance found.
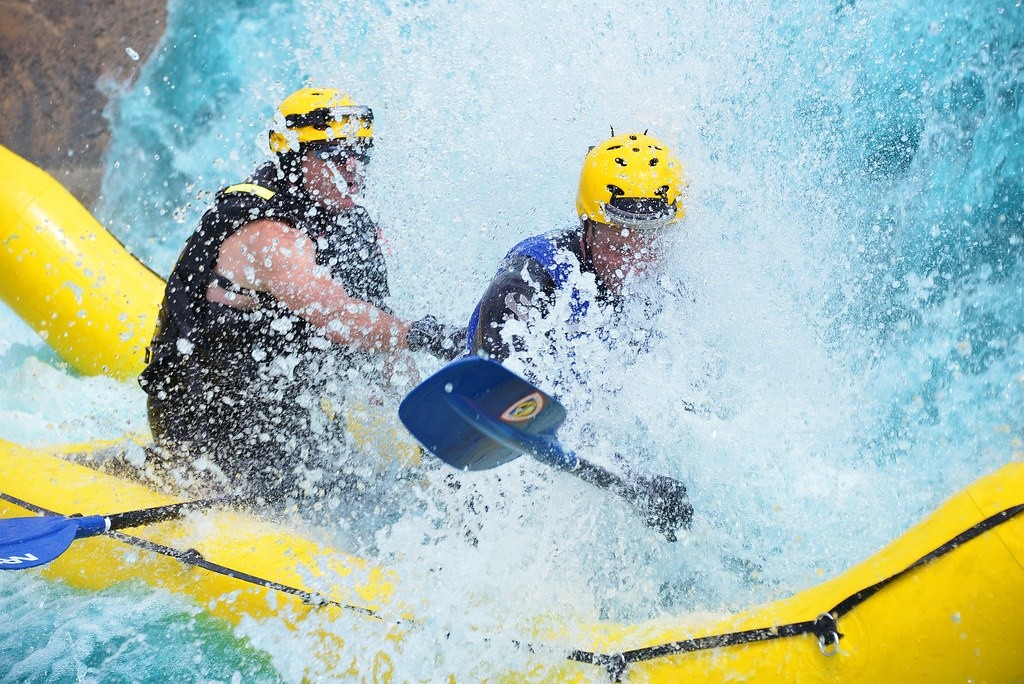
406, 314, 469, 362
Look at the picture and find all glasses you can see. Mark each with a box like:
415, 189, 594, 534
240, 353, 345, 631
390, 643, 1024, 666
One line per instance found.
312, 144, 370, 166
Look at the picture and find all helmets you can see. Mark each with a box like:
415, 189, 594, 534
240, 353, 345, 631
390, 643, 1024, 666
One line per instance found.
269, 87, 374, 154
576, 124, 692, 230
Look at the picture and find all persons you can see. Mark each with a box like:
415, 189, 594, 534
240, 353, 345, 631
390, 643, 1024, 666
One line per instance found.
467, 134, 683, 360
144, 88, 466, 490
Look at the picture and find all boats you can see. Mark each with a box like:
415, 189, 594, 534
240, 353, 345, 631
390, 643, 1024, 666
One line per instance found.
0, 144, 1024, 683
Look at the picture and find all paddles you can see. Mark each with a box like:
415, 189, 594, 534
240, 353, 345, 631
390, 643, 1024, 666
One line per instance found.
0, 492, 255, 574
395, 352, 767, 586
